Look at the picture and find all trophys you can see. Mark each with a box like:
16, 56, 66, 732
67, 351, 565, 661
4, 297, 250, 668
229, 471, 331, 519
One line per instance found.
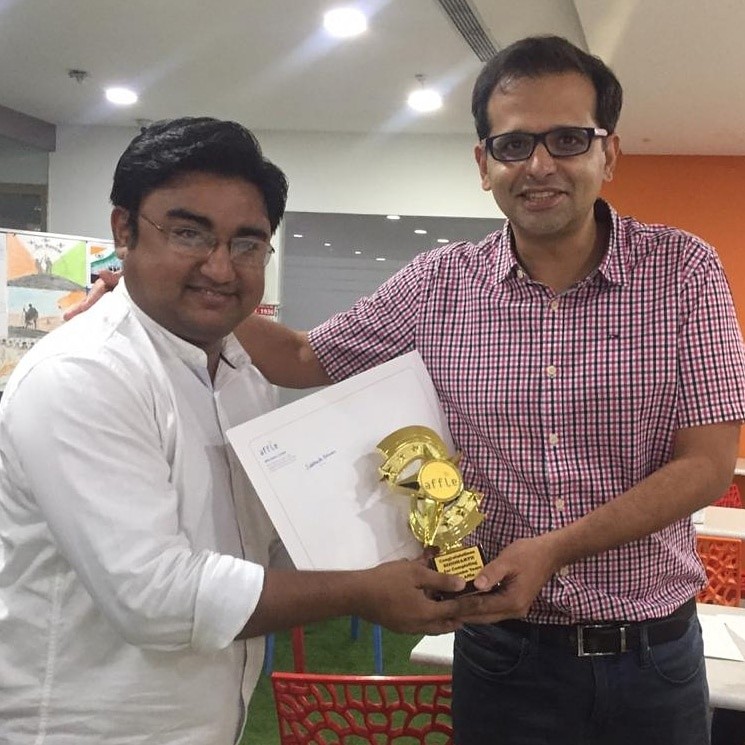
377, 425, 495, 602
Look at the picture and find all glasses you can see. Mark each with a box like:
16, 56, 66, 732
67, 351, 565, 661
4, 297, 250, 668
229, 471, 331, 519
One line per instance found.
481, 126, 610, 164
133, 212, 277, 269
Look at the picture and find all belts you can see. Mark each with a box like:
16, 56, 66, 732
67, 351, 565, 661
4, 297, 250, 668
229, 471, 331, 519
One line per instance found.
489, 596, 700, 661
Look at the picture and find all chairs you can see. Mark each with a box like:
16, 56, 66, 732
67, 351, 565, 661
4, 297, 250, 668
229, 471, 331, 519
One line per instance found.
695, 538, 741, 607
271, 672, 454, 745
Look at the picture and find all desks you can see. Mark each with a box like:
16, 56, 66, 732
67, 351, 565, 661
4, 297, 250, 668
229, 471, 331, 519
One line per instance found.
692, 505, 745, 538
410, 604, 745, 712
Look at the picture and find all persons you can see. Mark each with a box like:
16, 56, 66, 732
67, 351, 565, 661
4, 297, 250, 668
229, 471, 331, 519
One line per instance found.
0, 117, 481, 745
63, 35, 745, 745
